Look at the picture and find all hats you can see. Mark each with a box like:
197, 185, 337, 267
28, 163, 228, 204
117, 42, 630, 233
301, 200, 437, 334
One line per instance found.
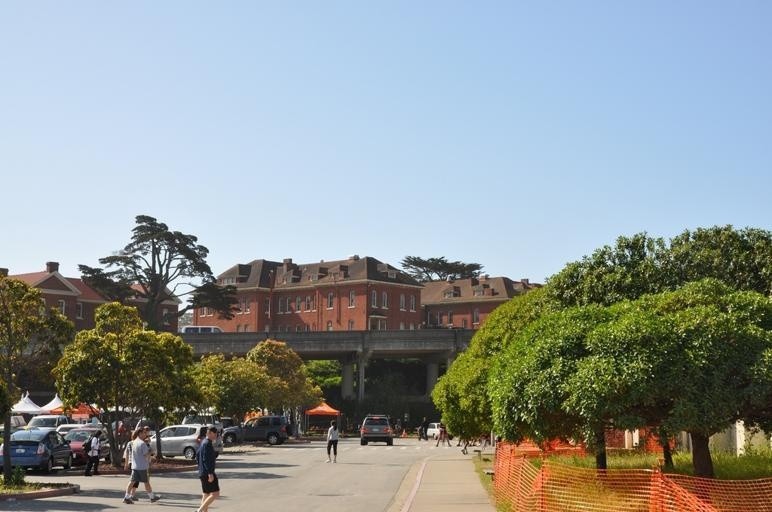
206, 426, 217, 433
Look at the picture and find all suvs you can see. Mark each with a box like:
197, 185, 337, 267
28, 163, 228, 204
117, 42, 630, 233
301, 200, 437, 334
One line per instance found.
358, 413, 395, 447
222, 415, 295, 447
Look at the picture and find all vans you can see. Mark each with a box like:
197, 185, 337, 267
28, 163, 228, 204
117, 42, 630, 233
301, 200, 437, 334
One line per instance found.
181, 325, 225, 334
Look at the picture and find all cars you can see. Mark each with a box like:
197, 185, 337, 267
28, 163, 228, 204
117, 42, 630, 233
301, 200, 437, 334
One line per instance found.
1, 405, 227, 476
426, 423, 455, 440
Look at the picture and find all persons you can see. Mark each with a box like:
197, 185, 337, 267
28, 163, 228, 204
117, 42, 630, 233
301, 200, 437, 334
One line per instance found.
84, 430, 103, 476
324, 419, 339, 463
386, 412, 489, 455
194, 425, 221, 512
122, 425, 163, 504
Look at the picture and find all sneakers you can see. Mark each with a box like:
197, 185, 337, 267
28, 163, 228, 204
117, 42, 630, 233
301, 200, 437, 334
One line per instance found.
122, 496, 138, 504
150, 495, 161, 502
326, 459, 336, 463
86, 472, 100, 476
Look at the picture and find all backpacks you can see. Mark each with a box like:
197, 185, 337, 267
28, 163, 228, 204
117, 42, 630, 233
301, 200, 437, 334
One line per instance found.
82, 437, 94, 454
